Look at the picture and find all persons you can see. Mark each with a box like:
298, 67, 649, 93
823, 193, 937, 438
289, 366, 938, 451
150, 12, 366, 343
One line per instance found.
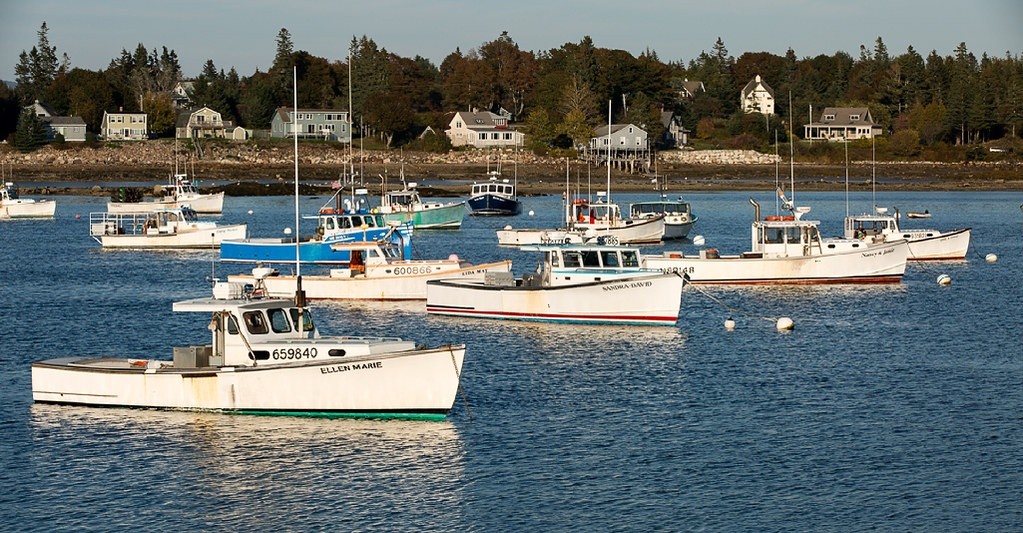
856, 229, 864, 240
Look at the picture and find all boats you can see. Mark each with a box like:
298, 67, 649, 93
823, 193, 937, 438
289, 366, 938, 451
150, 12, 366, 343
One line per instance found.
424, 98, 684, 327
219, 49, 415, 264
641, 90, 908, 285
822, 127, 972, 260
89, 134, 247, 248
629, 151, 699, 238
228, 223, 513, 302
906, 209, 931, 218
31, 65, 466, 421
0, 160, 56, 219
496, 155, 667, 247
106, 127, 225, 214
468, 128, 522, 217
320, 114, 466, 229
